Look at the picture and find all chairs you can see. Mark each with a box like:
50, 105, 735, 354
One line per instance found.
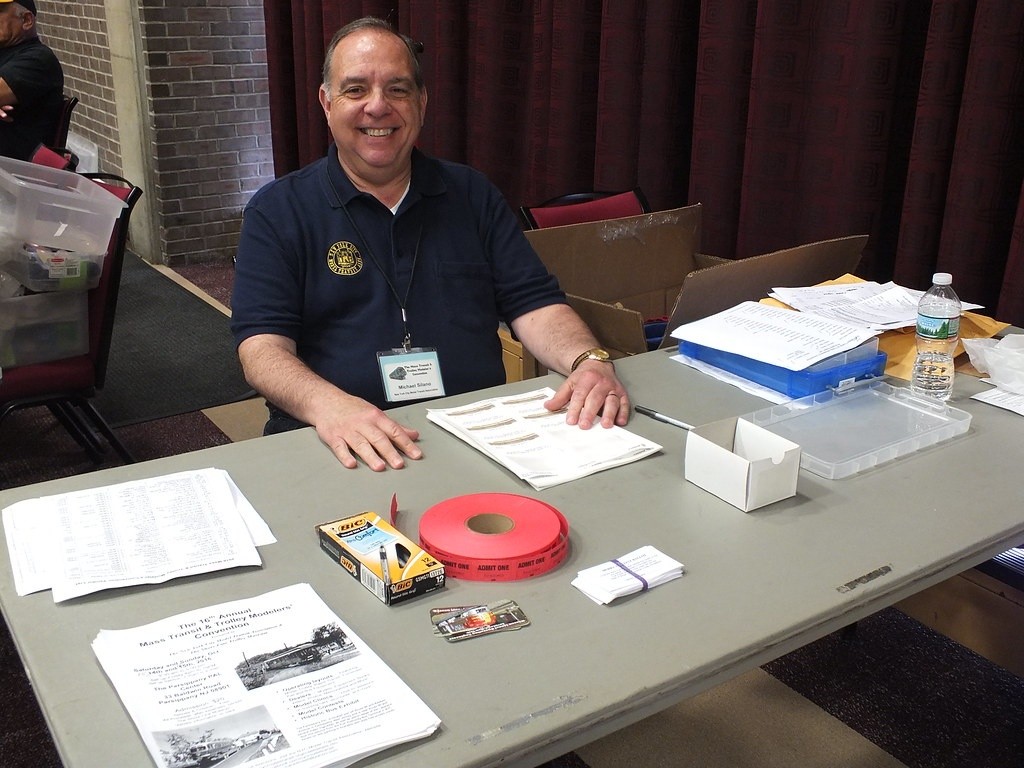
28, 94, 80, 169
0, 171, 142, 465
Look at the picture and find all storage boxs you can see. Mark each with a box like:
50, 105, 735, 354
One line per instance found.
313, 512, 447, 606
675, 338, 973, 478
0, 156, 128, 369
524, 203, 870, 372
685, 417, 801, 514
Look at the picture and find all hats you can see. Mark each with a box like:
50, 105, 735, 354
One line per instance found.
0, 0, 37, 16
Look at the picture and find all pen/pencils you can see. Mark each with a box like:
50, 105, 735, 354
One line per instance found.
380, 546, 393, 585
635, 405, 694, 430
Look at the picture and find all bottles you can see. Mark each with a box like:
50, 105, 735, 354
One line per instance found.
911, 273, 961, 401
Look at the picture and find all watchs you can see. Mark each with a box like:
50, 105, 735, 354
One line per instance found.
571, 348, 615, 373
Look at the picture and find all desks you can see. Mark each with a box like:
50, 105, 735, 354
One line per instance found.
0, 275, 1024, 768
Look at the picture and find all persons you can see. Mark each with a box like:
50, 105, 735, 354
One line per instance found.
0, 0, 64, 160
229, 17, 630, 473
